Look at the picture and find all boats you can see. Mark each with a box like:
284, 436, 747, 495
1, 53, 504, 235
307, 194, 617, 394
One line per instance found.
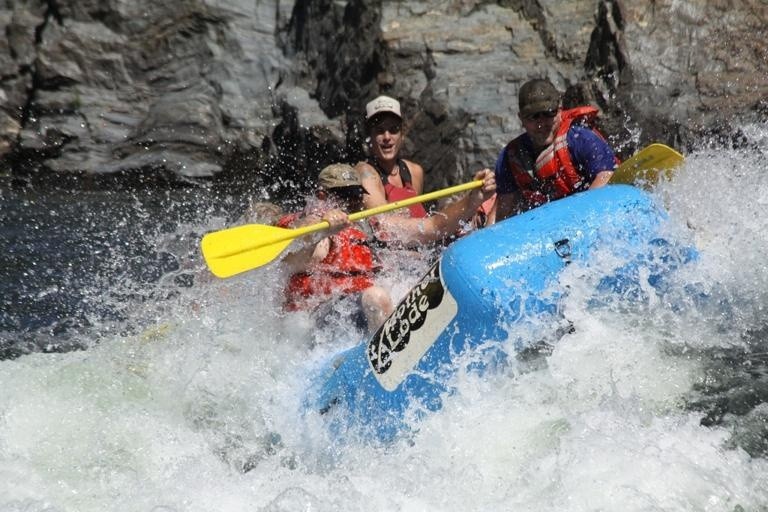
296, 182, 729, 449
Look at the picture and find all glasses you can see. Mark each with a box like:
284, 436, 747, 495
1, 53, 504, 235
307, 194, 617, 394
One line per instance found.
527, 110, 557, 121
375, 123, 401, 134
335, 190, 362, 199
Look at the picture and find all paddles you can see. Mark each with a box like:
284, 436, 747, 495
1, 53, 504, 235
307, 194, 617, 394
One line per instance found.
201, 170, 491, 277
606, 143, 686, 189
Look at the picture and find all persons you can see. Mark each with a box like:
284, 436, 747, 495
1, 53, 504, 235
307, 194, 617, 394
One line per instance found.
276, 162, 497, 343
486, 77, 615, 228
360, 94, 432, 248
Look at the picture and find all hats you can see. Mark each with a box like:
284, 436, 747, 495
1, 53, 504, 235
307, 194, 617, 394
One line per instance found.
319, 163, 370, 195
519, 79, 560, 119
365, 96, 401, 120
